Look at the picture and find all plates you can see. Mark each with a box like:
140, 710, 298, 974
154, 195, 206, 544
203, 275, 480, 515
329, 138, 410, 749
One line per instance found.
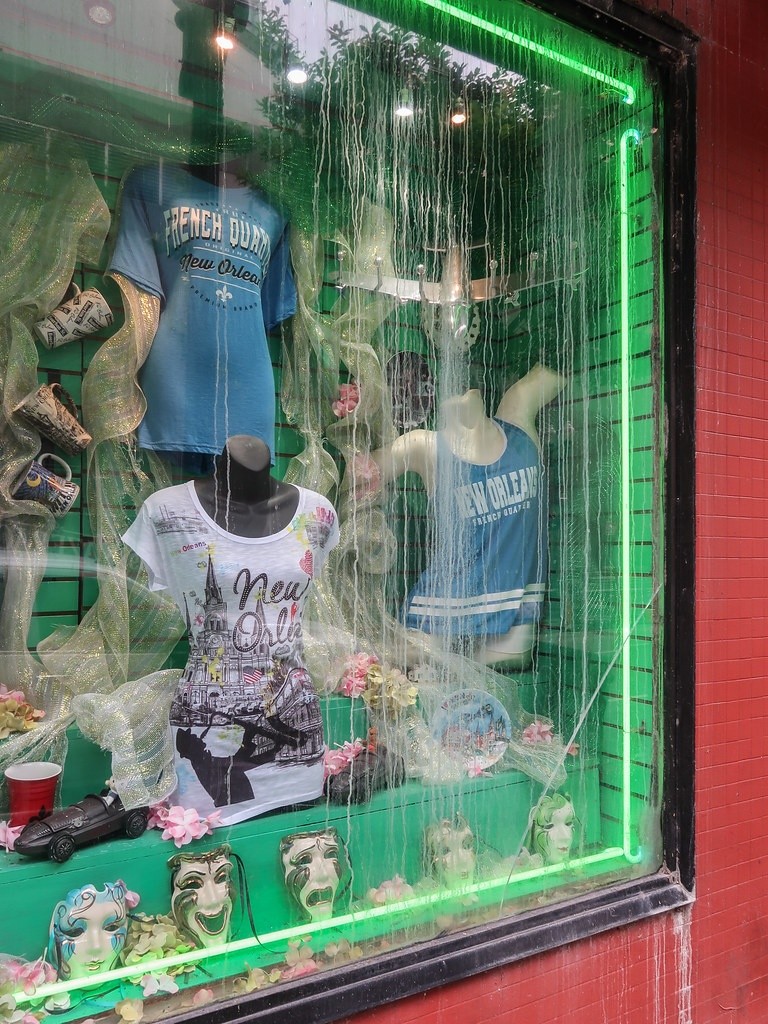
431, 688, 512, 770
382, 351, 435, 427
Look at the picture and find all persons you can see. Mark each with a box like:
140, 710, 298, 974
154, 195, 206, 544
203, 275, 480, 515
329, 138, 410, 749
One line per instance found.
120, 437, 343, 835
107, 155, 300, 469
367, 359, 569, 672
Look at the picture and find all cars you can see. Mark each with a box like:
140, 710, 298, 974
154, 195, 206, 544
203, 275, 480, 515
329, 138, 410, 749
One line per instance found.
13, 788, 150, 864
324, 742, 405, 806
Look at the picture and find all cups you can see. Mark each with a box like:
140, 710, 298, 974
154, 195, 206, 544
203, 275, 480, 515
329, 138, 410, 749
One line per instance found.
11, 453, 80, 519
33, 280, 117, 349
4, 761, 62, 830
11, 383, 91, 456
